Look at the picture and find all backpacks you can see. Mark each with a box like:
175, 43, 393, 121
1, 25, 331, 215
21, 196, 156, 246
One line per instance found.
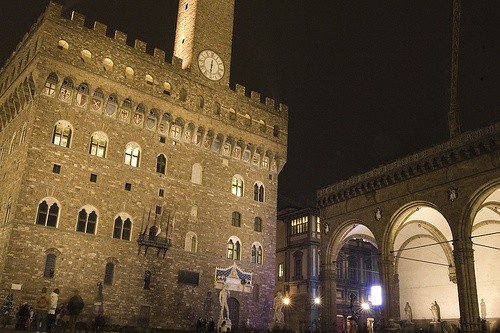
37, 294, 48, 308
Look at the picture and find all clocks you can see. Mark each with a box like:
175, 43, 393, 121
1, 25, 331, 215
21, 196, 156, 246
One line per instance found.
198, 50, 225, 81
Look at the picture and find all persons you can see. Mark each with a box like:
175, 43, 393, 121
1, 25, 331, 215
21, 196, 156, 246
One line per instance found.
219, 286, 230, 320
67, 289, 84, 333
404, 302, 412, 322
92, 314, 105, 333
430, 303, 438, 321
97, 281, 103, 295
273, 292, 284, 322
195, 316, 229, 333
15, 286, 60, 333
